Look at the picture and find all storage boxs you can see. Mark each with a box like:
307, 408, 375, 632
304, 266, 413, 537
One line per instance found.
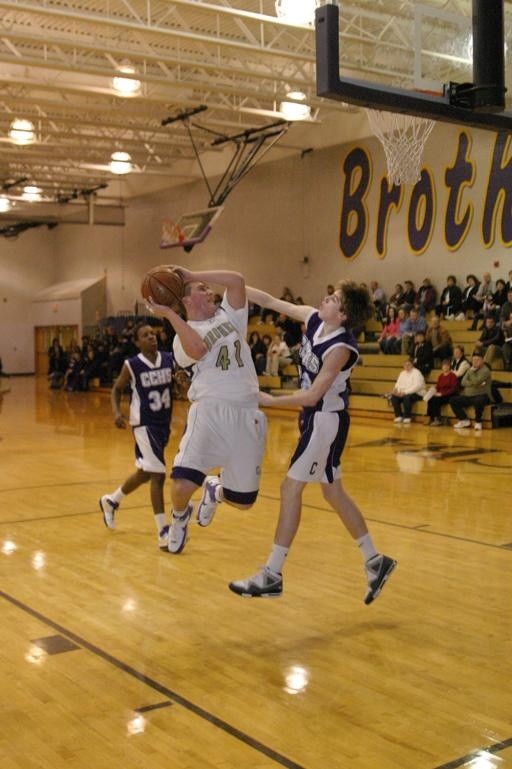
248, 311, 512, 431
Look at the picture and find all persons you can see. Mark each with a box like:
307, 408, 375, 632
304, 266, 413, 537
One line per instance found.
248, 285, 333, 376
360, 276, 510, 382
449, 352, 494, 428
391, 359, 426, 422
48, 294, 223, 391
143, 265, 267, 554
227, 281, 398, 606
2, 359, 14, 382
99, 322, 192, 551
423, 360, 459, 426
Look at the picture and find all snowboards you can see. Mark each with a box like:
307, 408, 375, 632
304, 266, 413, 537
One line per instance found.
141, 267, 183, 309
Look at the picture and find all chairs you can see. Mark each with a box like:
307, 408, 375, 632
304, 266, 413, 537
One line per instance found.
444, 313, 466, 321
454, 419, 471, 428
474, 423, 483, 430
394, 417, 412, 423
229, 567, 283, 598
197, 476, 219, 527
159, 502, 193, 554
424, 417, 444, 426
100, 496, 118, 531
364, 554, 397, 605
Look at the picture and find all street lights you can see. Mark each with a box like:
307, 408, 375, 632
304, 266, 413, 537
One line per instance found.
491, 382, 503, 403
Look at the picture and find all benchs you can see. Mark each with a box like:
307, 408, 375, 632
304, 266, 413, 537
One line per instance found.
248, 311, 512, 431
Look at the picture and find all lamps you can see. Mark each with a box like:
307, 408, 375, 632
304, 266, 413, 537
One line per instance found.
1, 2, 321, 213
